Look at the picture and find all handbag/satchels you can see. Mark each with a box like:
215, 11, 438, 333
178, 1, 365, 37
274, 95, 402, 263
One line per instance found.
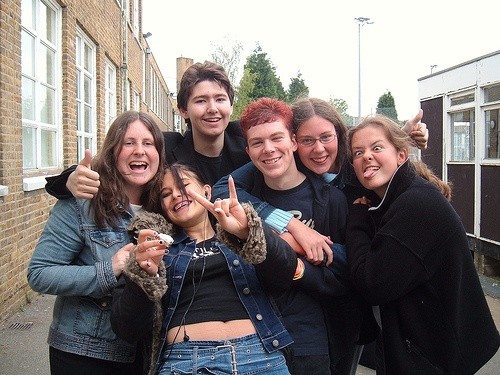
111, 271, 155, 344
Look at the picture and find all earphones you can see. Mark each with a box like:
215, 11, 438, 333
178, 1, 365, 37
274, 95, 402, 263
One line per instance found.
183, 331, 189, 342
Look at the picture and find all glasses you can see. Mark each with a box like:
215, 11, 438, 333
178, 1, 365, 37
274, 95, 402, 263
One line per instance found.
296, 134, 338, 147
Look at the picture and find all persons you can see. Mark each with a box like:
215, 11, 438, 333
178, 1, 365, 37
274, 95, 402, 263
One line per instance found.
134, 160, 298, 375
209, 97, 500, 375
45, 61, 429, 201
25, 110, 165, 375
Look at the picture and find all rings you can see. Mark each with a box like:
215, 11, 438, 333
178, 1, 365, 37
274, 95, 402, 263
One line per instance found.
215, 198, 221, 201
147, 260, 151, 269
